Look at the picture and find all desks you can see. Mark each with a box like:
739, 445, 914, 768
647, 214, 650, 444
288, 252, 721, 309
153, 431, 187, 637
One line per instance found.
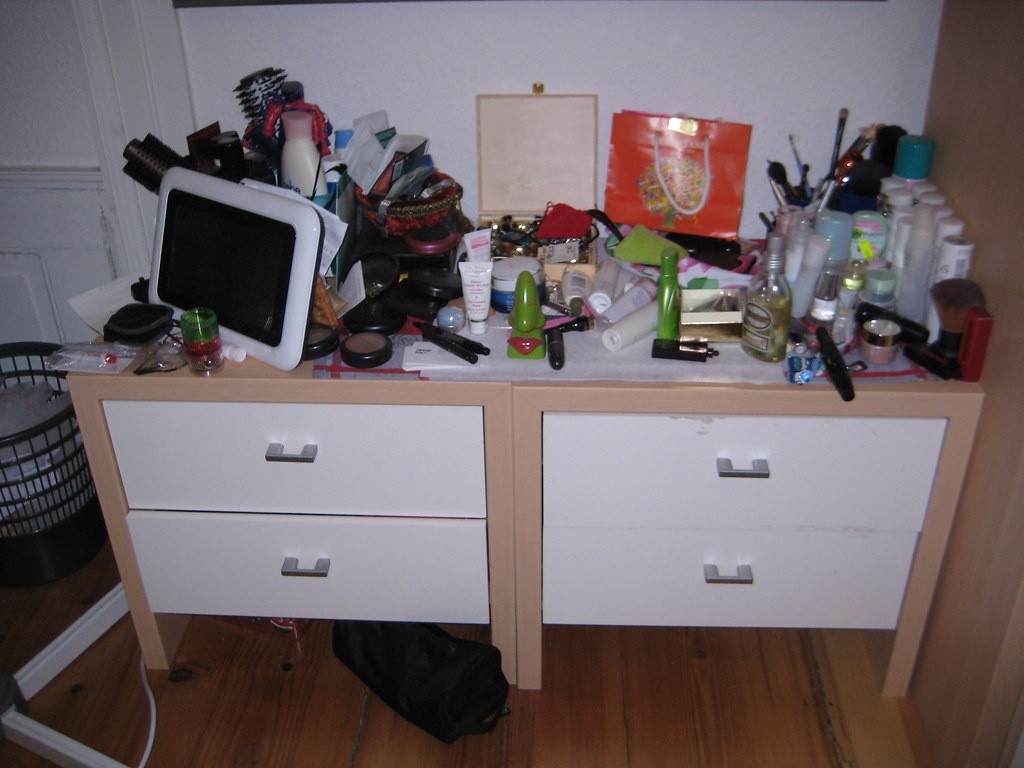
65, 235, 986, 699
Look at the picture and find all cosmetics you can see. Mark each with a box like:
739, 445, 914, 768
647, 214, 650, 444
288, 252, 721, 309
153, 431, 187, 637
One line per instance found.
740, 108, 986, 401
340, 331, 395, 368
490, 225, 757, 360
651, 338, 719, 363
45, 66, 490, 375
458, 260, 493, 335
464, 227, 492, 262
302, 323, 338, 361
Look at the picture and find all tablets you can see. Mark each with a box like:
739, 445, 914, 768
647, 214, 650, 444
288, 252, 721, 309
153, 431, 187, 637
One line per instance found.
148, 166, 326, 372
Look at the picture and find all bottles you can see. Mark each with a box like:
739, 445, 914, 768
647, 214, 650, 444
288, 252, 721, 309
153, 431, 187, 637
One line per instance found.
741, 249, 791, 363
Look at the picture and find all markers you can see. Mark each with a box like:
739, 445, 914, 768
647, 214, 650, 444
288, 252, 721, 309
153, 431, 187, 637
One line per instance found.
422, 329, 479, 365
412, 320, 491, 356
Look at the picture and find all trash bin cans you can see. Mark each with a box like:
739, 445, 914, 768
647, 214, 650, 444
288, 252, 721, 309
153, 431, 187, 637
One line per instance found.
0, 341, 112, 590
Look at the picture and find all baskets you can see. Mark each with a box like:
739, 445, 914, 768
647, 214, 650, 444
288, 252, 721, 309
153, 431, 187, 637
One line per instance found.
0, 341, 106, 586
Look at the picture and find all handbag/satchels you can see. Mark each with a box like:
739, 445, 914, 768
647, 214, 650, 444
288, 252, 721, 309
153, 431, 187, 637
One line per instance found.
332, 619, 510, 745
601, 108, 753, 243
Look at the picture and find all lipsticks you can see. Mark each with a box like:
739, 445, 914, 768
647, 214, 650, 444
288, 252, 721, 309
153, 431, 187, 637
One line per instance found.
856, 302, 930, 344
957, 306, 993, 382
904, 343, 961, 379
547, 329, 564, 370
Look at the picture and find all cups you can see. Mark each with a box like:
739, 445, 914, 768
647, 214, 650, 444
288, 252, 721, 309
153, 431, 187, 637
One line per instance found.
180, 307, 224, 377
